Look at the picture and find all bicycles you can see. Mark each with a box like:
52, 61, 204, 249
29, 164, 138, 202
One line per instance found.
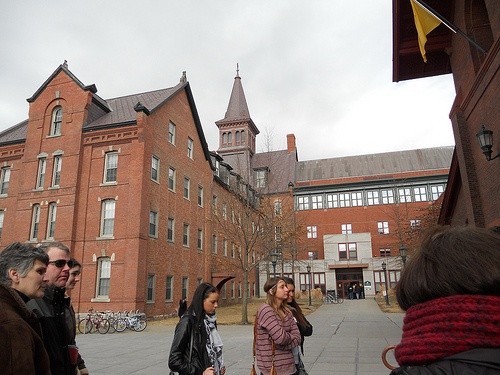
322, 290, 344, 304
77, 307, 147, 334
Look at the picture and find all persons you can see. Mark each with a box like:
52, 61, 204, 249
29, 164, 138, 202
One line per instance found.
64, 257, 90, 375
348, 283, 363, 300
388, 224, 500, 375
168, 282, 225, 375
26, 242, 76, 375
279, 277, 312, 375
178, 296, 187, 319
251, 276, 301, 375
0, 242, 49, 375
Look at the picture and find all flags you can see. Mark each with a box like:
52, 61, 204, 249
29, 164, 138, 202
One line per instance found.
410, 0, 441, 63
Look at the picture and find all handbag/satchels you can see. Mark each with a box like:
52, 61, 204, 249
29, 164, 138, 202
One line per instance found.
250, 364, 277, 375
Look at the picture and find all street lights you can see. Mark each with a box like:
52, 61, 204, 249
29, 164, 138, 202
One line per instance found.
306, 263, 312, 305
270, 250, 279, 278
399, 244, 408, 270
381, 261, 390, 306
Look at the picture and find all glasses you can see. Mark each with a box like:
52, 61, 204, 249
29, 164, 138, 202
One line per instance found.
49, 259, 74, 268
